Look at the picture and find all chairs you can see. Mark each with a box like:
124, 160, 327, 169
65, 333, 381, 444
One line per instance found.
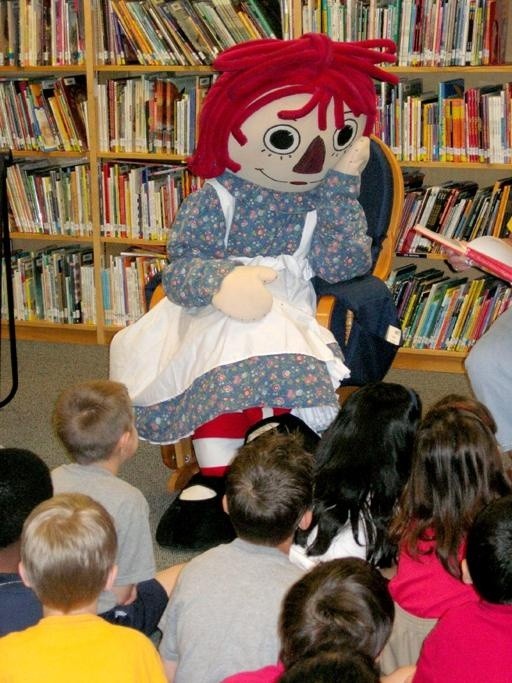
145, 134, 402, 492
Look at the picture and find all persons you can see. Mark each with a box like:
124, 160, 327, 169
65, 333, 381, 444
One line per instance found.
111, 32, 401, 550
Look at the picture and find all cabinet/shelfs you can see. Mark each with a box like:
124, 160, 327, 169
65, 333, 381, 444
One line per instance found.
0, 1, 99, 345
297, 0, 512, 373
89, 0, 297, 345
